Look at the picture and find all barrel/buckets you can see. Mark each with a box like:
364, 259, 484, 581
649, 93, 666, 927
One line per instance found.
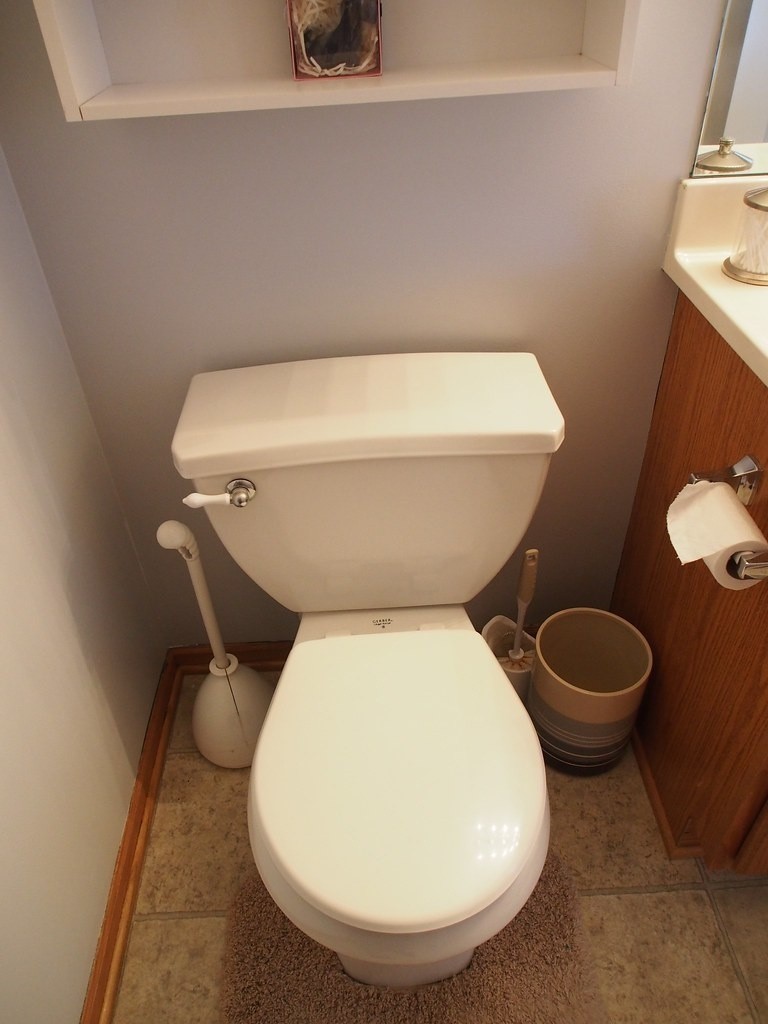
524, 609, 654, 776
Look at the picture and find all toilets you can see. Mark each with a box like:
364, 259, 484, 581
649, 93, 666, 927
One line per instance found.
167, 352, 566, 993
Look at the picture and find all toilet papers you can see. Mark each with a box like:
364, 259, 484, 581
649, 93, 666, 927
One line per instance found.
665, 480, 768, 590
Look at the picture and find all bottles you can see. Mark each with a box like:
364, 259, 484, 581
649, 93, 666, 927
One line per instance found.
722, 188, 768, 286
690, 137, 753, 175
